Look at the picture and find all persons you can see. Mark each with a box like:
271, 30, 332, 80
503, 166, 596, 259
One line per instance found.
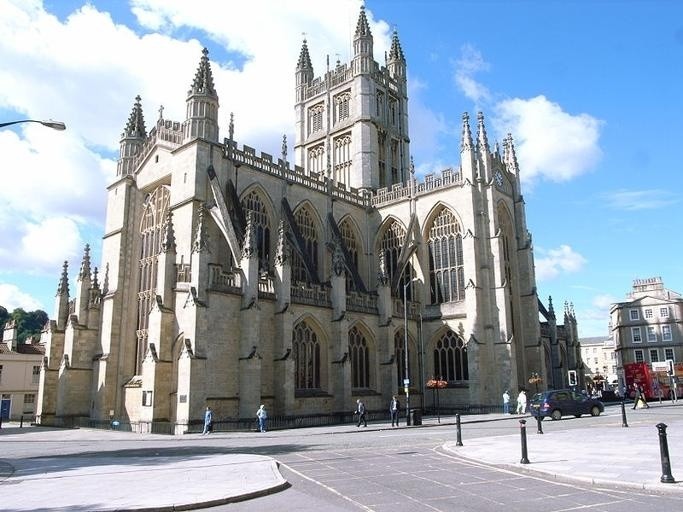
256, 404, 270, 433
517, 390, 528, 414
390, 396, 401, 428
354, 399, 369, 428
630, 382, 650, 409
201, 406, 216, 435
503, 389, 513, 415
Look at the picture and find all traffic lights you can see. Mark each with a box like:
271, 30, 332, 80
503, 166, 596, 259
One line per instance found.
570, 372, 575, 384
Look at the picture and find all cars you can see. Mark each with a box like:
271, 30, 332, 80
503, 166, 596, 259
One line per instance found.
529, 389, 604, 420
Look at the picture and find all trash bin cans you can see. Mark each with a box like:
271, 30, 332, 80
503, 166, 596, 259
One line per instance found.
410, 409, 422, 425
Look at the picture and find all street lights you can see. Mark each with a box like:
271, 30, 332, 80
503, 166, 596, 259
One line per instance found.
0, 120, 66, 130
404, 277, 421, 426
